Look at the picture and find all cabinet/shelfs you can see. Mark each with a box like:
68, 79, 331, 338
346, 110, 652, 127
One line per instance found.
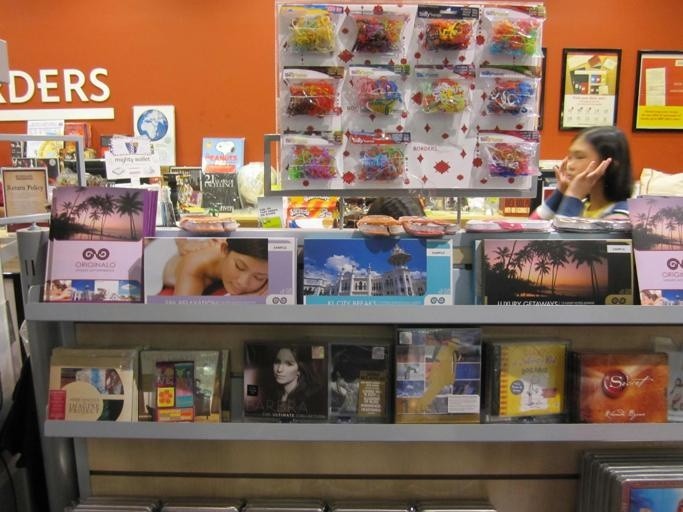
14, 228, 682, 512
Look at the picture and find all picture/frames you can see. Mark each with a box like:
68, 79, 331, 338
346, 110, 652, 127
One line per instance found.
632, 49, 683, 133
559, 47, 622, 132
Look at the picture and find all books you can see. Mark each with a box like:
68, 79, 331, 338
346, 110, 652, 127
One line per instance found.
68, 495, 495, 510
579, 445, 682, 512
0, 105, 683, 424
